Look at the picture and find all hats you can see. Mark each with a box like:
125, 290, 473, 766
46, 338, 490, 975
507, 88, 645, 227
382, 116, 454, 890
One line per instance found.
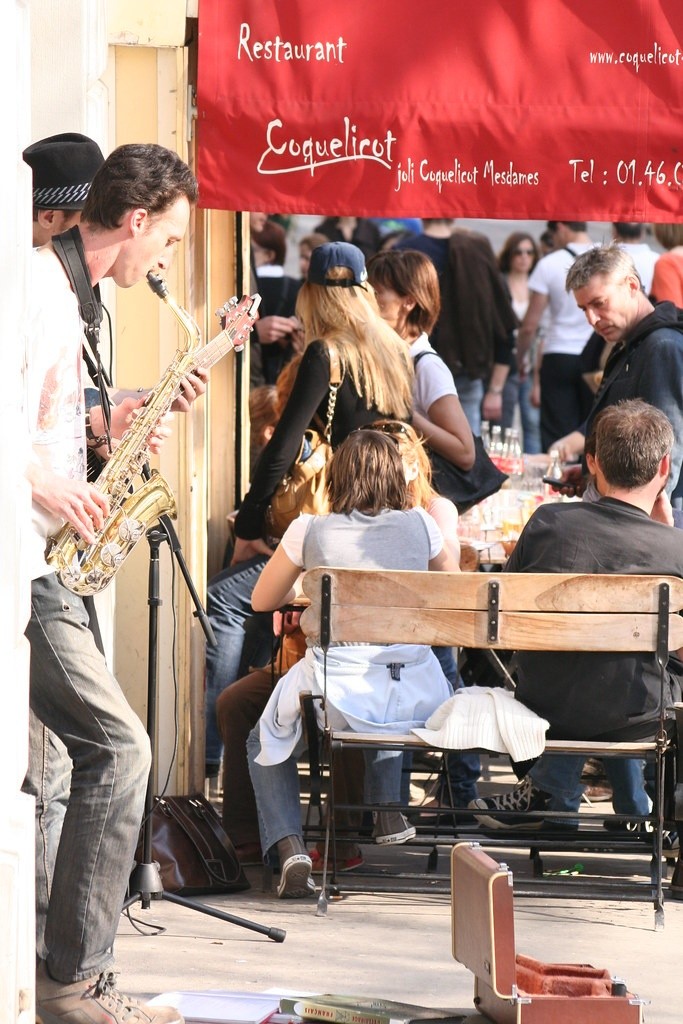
22, 132, 105, 211
308, 242, 369, 293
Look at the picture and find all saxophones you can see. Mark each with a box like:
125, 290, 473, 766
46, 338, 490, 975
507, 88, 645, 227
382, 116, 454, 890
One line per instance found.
44, 269, 204, 598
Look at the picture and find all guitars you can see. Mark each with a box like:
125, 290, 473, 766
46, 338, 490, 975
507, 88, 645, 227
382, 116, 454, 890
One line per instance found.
84, 293, 263, 500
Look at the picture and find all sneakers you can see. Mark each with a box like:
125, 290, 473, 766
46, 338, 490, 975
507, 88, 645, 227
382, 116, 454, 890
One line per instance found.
276, 834, 316, 899
372, 802, 416, 846
408, 774, 683, 900
36, 959, 185, 1024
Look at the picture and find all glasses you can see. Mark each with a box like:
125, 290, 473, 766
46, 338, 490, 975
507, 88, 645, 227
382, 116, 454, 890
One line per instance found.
511, 249, 535, 255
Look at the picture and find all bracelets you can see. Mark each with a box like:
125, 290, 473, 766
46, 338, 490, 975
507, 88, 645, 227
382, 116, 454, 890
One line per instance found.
488, 388, 503, 394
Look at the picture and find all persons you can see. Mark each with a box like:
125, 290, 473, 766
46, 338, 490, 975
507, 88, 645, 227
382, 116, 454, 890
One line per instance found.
204, 213, 683, 901
31, 143, 212, 1024
24, 134, 107, 949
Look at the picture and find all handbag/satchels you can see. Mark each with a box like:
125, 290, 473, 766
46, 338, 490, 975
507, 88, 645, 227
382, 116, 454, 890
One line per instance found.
261, 338, 342, 540
133, 792, 252, 896
411, 351, 510, 517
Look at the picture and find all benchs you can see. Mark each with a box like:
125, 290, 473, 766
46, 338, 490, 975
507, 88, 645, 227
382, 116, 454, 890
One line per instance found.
296, 566, 682, 913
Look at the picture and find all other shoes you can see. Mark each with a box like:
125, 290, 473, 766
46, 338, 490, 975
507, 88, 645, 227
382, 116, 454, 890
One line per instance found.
309, 849, 365, 874
234, 842, 263, 866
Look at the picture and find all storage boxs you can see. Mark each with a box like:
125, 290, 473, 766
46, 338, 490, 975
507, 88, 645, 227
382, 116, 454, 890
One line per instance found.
453, 839, 641, 1023
